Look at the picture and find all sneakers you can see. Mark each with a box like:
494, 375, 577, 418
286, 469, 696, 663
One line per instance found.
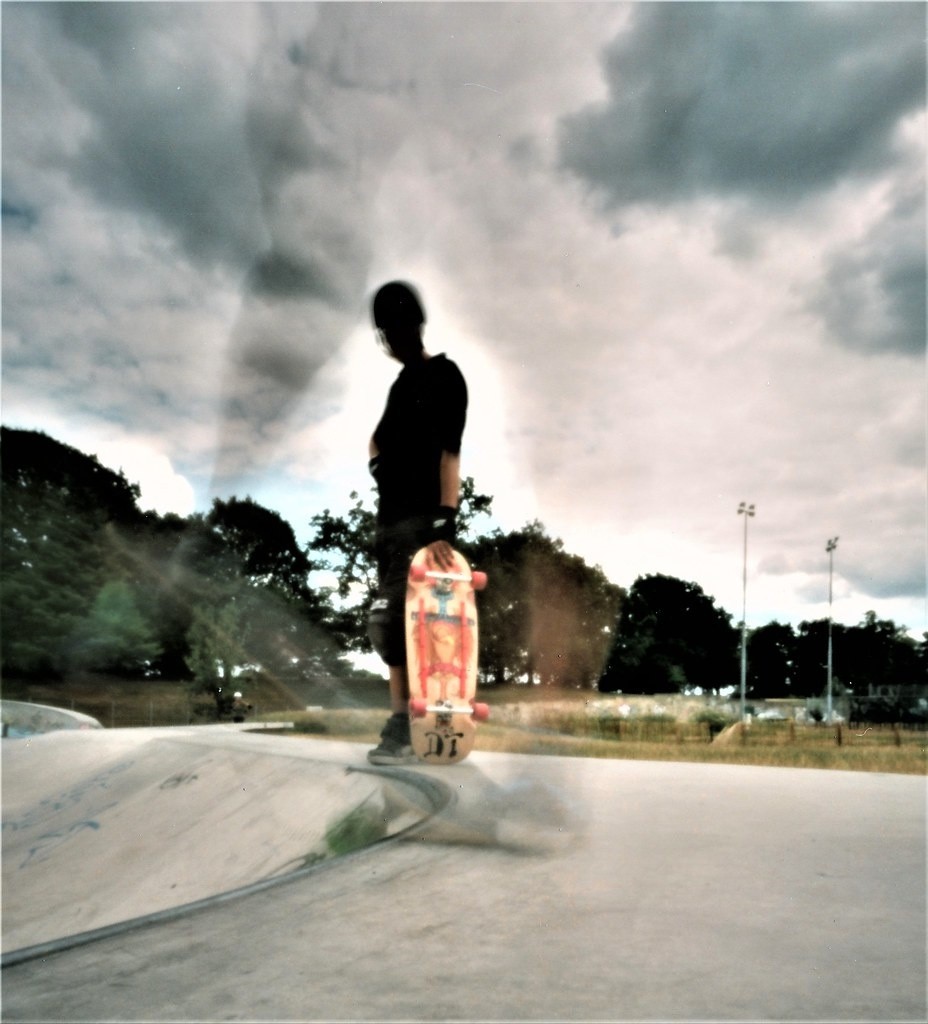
368, 713, 419, 765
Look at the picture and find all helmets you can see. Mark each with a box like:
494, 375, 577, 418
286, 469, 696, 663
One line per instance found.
373, 283, 424, 328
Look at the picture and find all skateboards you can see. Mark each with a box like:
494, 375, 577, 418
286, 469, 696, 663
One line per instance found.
404, 542, 490, 766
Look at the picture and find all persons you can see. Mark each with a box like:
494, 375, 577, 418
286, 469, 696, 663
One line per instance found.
368, 281, 468, 764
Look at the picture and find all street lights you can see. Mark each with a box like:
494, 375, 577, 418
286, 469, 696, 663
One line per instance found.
826, 537, 839, 727
737, 502, 755, 721
